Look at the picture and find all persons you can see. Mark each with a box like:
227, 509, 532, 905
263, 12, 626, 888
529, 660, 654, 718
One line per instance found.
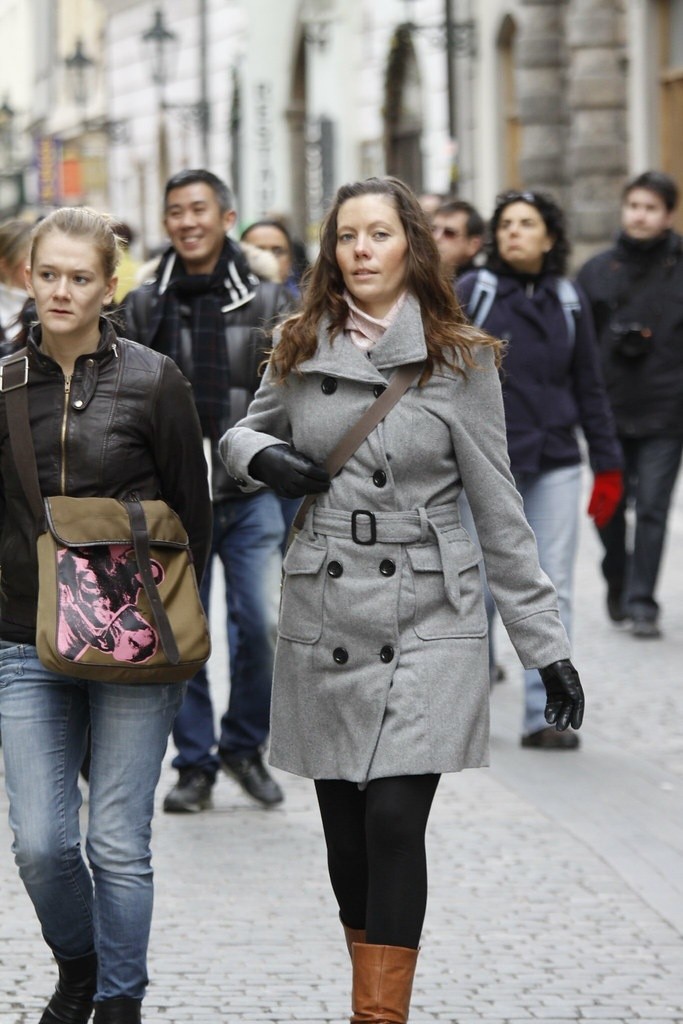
577, 171, 683, 631
241, 222, 312, 300
417, 192, 442, 216
220, 175, 589, 1024
0, 206, 215, 1024
0, 221, 38, 357
431, 201, 487, 280
117, 168, 291, 810
108, 223, 139, 309
449, 189, 625, 750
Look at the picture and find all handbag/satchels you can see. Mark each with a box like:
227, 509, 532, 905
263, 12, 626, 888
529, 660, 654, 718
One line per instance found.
35, 492, 210, 682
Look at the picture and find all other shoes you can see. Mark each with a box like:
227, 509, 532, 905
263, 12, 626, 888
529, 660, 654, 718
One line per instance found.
631, 614, 661, 638
608, 557, 633, 622
521, 724, 578, 751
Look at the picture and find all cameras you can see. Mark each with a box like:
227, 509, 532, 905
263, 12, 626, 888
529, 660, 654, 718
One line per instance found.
611, 322, 652, 357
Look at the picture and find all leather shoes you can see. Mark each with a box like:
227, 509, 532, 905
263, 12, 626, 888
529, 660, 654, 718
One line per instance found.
218, 744, 283, 803
163, 758, 215, 813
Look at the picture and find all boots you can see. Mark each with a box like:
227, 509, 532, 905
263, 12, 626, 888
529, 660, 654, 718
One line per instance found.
37, 946, 100, 1024
93, 993, 145, 1024
336, 911, 419, 1024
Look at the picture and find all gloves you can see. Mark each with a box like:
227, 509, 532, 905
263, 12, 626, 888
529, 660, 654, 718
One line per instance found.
586, 471, 623, 529
539, 659, 583, 733
247, 446, 330, 500
